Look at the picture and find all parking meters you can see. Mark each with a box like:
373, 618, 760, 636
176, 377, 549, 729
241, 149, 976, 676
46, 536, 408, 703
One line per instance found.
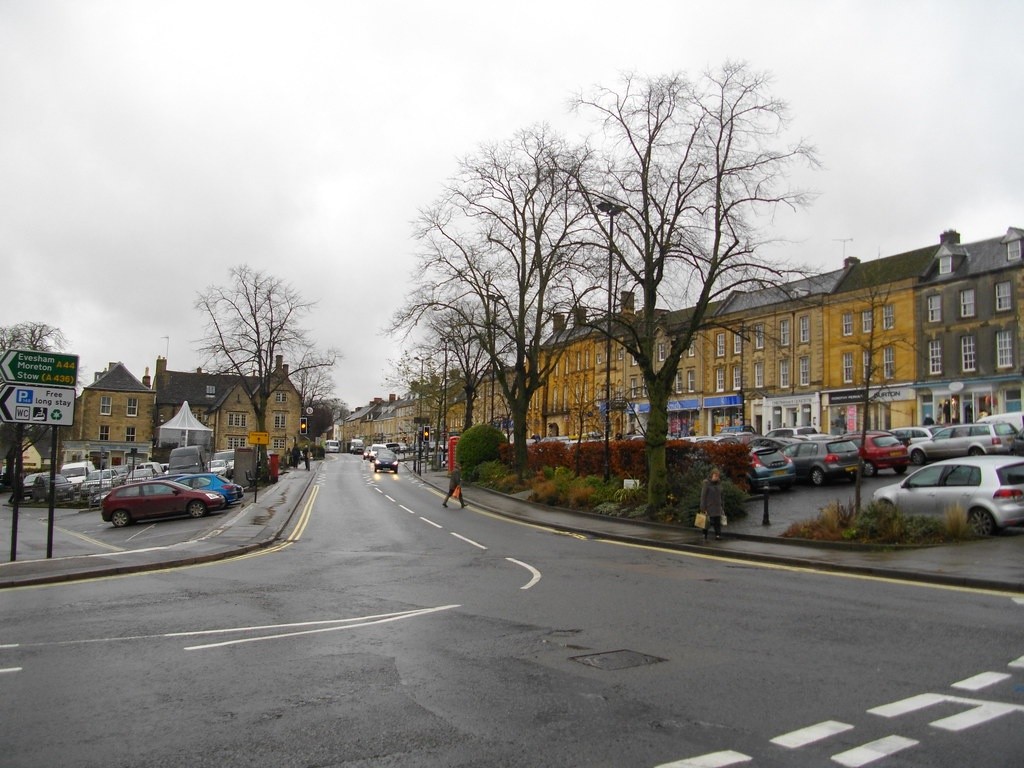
758, 480, 771, 527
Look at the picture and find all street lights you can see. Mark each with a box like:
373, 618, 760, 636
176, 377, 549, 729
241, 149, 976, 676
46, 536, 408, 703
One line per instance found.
415, 357, 430, 478
440, 337, 454, 467
595, 203, 629, 482
486, 295, 506, 426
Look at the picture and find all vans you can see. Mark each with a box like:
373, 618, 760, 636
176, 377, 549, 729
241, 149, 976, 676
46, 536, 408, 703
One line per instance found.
169, 445, 206, 474
975, 411, 1024, 439
765, 426, 819, 438
720, 426, 756, 434
350, 438, 363, 454
324, 440, 340, 453
62, 460, 95, 492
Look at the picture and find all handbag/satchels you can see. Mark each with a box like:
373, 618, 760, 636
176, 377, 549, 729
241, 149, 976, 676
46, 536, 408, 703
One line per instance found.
452, 486, 460, 498
721, 510, 727, 526
695, 510, 711, 529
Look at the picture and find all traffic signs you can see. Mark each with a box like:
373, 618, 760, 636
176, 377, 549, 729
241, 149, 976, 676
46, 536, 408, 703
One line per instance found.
0, 349, 79, 426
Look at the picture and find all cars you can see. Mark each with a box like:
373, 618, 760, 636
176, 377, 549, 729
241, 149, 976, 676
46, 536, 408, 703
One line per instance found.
354, 444, 365, 455
23, 472, 76, 502
842, 422, 1024, 536
739, 434, 866, 493
0, 465, 16, 484
81, 460, 244, 528
364, 443, 399, 474
526, 434, 646, 450
677, 432, 755, 445
387, 443, 408, 454
208, 449, 235, 479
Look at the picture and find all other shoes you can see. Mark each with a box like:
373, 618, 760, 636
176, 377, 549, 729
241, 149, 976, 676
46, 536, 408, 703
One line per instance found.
442, 502, 448, 508
461, 504, 468, 508
703, 534, 708, 541
715, 535, 722, 540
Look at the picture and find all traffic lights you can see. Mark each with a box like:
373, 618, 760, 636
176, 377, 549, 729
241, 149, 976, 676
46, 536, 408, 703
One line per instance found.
423, 426, 430, 442
299, 418, 307, 435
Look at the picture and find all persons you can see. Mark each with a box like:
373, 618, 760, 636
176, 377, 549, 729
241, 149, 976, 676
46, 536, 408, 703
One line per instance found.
292, 444, 300, 469
303, 444, 308, 469
531, 433, 540, 439
443, 463, 468, 508
700, 468, 724, 542
287, 448, 291, 454
615, 431, 622, 440
924, 414, 934, 425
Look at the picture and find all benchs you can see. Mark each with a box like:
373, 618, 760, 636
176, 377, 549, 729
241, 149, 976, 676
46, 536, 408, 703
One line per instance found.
245, 470, 261, 488
